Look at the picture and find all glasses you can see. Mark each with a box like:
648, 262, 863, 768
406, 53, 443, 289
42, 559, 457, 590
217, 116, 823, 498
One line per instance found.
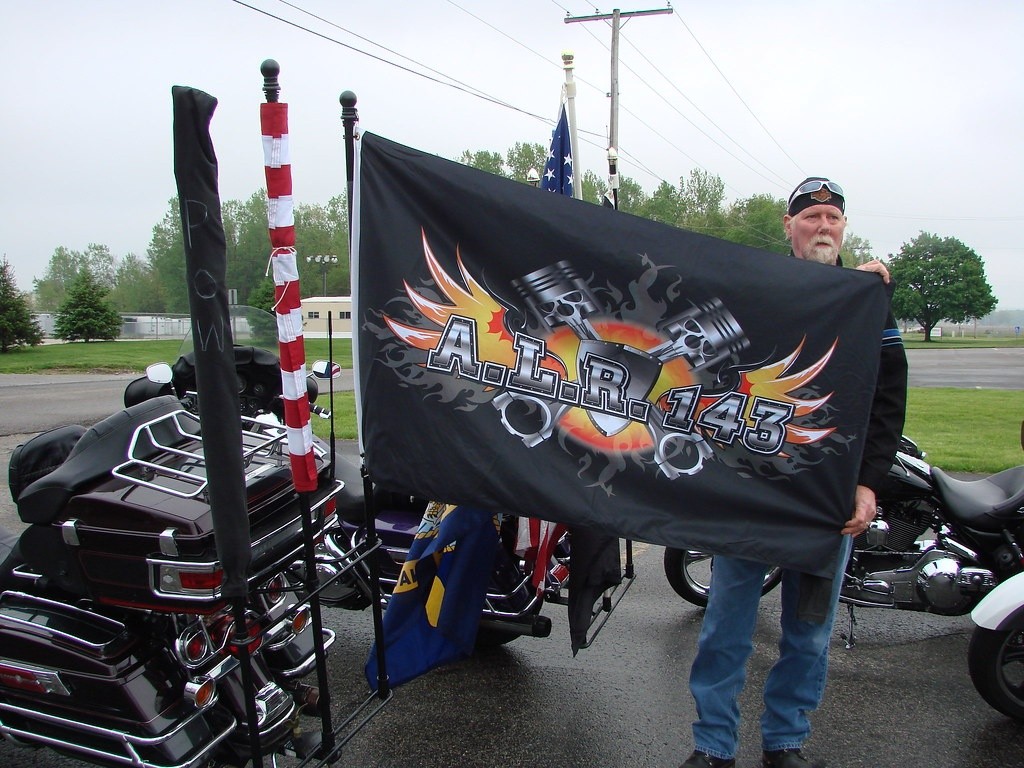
787, 181, 845, 219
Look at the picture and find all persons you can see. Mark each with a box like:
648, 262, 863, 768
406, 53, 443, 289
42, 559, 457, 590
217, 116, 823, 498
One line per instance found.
679, 178, 908, 766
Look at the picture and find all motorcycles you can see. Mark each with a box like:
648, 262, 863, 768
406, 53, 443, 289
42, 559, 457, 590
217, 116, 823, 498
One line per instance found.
664, 435, 1024, 650
0, 359, 637, 768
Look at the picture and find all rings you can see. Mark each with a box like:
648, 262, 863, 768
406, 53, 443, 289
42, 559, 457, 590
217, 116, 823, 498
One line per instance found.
866, 520, 870, 526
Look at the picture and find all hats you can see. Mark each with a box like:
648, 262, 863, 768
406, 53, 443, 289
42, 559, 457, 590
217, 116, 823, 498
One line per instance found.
788, 177, 845, 216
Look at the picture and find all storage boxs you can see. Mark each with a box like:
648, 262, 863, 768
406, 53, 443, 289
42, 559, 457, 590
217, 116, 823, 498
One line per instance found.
69, 431, 335, 601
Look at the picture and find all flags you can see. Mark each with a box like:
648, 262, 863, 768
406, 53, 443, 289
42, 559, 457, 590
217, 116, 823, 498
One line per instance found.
365, 502, 492, 690
498, 106, 623, 656
351, 129, 892, 576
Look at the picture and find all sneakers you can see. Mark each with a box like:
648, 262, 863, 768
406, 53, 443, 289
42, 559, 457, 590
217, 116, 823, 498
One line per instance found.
763, 747, 814, 768
679, 748, 735, 768
